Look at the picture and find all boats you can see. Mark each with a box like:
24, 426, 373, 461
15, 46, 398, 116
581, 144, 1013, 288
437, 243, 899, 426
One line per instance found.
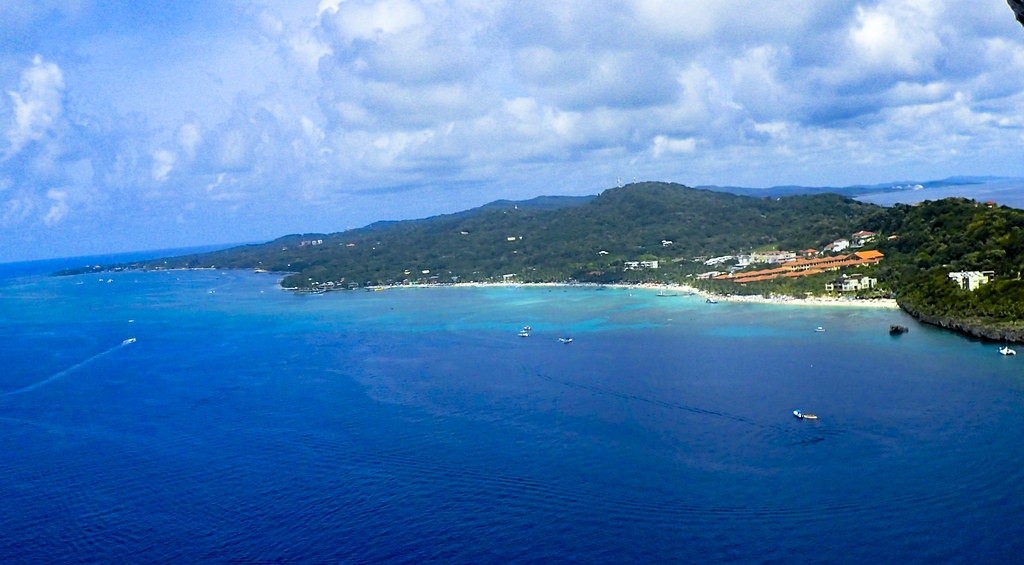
517, 326, 532, 337
793, 410, 818, 419
559, 337, 573, 343
